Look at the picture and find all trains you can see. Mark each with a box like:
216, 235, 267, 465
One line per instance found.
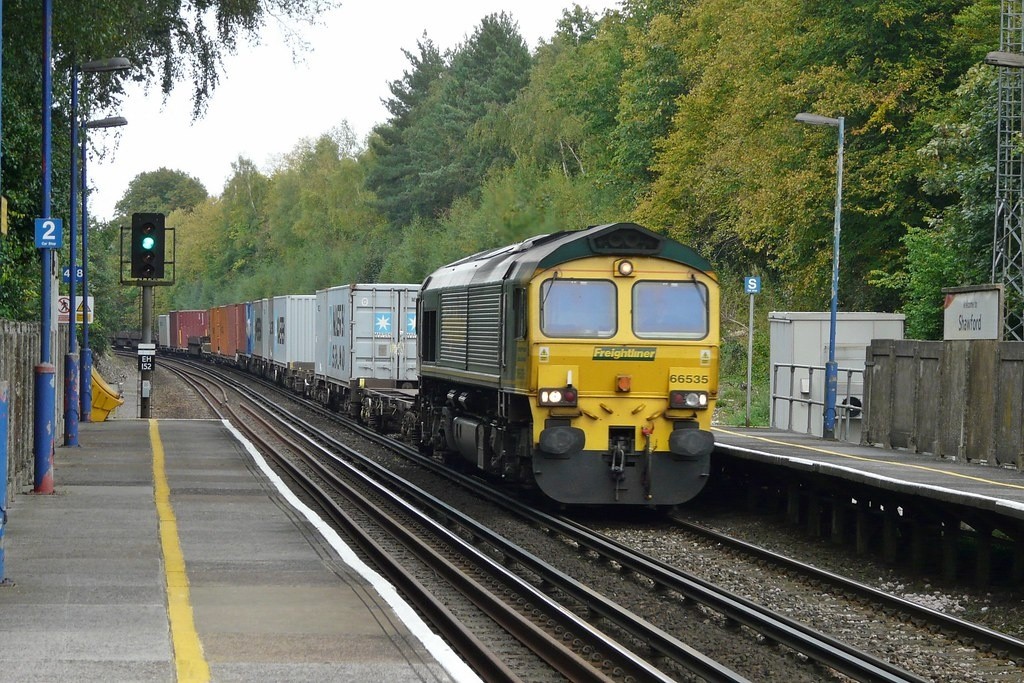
105, 222, 721, 505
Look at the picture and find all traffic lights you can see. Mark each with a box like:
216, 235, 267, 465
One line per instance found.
131, 212, 165, 278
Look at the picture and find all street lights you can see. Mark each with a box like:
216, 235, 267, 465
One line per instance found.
794, 113, 844, 441
80, 116, 128, 423
63, 57, 132, 447
